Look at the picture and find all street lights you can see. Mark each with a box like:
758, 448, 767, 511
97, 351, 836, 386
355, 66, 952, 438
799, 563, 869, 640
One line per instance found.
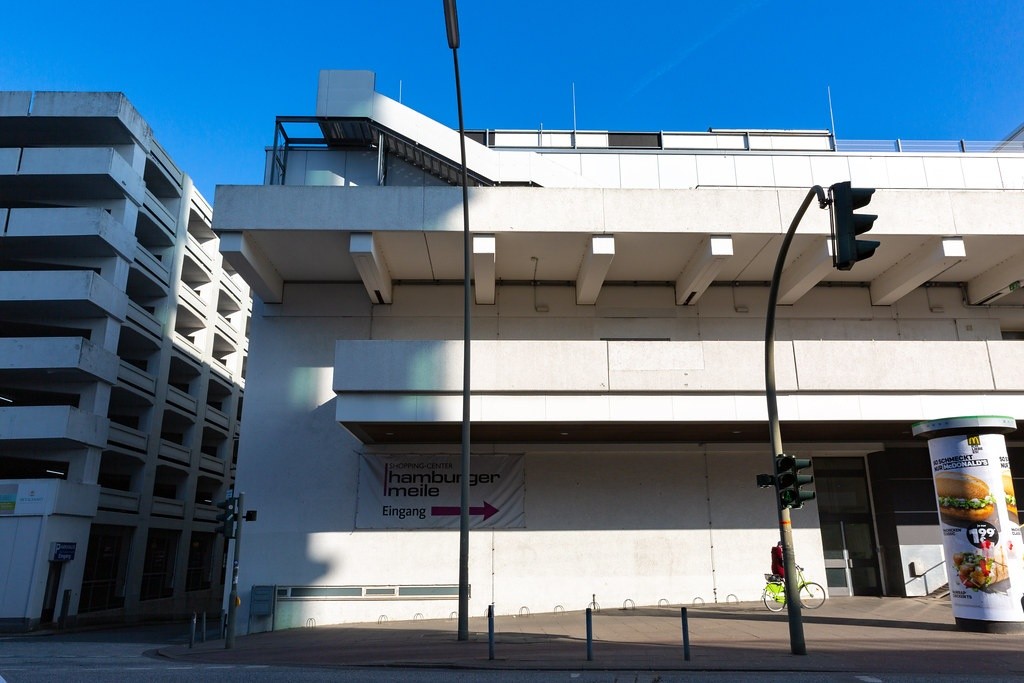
442, 1, 474, 641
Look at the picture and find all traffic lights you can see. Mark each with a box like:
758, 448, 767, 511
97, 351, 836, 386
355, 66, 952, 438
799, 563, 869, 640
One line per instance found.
214, 497, 236, 536
791, 456, 815, 508
775, 454, 799, 509
828, 181, 880, 271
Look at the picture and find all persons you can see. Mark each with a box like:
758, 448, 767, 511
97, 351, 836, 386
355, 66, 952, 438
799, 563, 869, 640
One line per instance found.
771, 540, 803, 580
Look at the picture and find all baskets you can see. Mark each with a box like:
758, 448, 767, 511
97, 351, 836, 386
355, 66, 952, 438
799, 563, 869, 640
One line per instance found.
765, 573, 781, 582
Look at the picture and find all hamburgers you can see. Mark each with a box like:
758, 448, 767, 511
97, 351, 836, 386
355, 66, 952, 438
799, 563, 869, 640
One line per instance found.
1003, 475, 1019, 524
935, 471, 995, 522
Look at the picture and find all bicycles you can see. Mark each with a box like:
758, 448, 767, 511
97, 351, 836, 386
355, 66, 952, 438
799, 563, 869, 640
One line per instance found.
763, 563, 826, 612
